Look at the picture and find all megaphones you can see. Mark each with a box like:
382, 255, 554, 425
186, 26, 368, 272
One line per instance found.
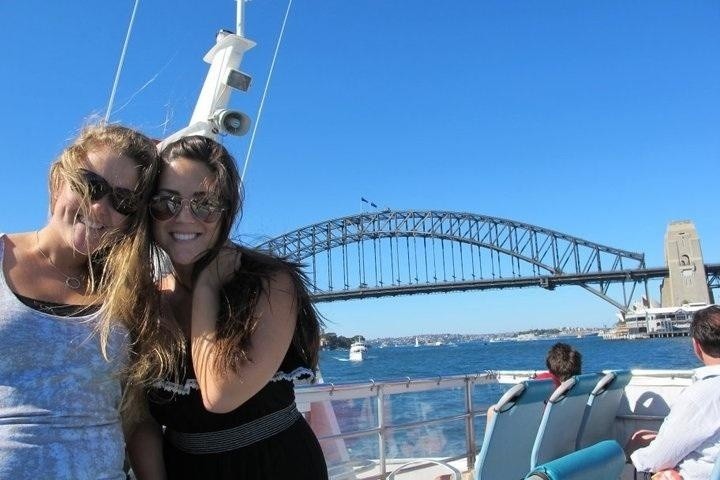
208, 109, 251, 136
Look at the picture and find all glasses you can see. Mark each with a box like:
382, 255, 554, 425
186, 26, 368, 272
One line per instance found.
149, 195, 223, 222
70, 168, 143, 216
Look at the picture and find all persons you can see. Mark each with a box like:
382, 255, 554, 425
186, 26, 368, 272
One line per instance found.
120, 135, 328, 480
547, 342, 582, 385
0, 124, 186, 480
627, 304, 720, 480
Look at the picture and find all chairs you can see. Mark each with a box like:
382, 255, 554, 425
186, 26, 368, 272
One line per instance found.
530, 373, 606, 468
524, 439, 627, 477
388, 378, 555, 479
576, 369, 634, 455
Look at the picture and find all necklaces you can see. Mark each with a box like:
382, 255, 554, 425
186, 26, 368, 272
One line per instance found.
35, 230, 80, 289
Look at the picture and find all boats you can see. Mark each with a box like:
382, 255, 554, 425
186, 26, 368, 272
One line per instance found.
349, 336, 369, 362
293, 365, 696, 479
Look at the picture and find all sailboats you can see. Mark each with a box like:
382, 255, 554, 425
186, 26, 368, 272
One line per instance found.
414, 337, 421, 348
576, 329, 583, 339
